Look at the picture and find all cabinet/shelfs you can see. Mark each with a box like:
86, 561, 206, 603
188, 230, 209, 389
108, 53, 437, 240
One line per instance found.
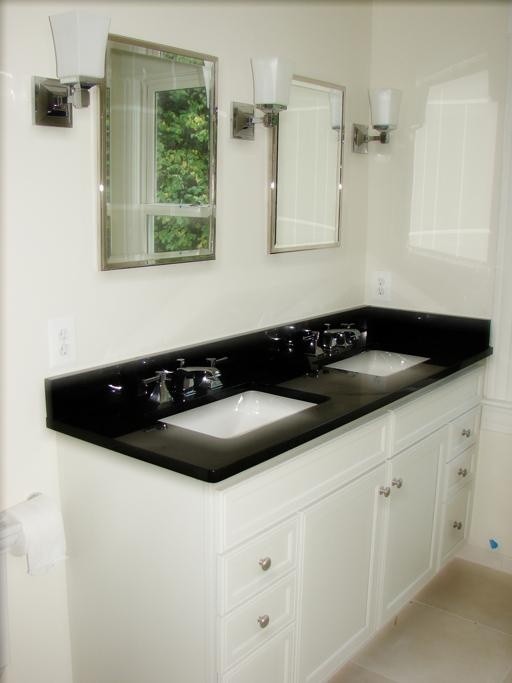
54, 359, 486, 683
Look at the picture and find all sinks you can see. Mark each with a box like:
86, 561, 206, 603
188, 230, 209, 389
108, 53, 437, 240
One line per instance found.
157, 390, 330, 439
321, 351, 431, 377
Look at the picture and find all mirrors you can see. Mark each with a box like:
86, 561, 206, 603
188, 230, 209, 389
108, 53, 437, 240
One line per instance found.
267, 73, 346, 256
99, 32, 220, 271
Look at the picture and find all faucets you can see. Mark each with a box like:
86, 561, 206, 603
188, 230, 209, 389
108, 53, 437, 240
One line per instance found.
177, 365, 219, 391
323, 328, 363, 352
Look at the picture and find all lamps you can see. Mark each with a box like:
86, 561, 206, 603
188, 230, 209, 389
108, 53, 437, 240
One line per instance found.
352, 88, 402, 154
232, 56, 294, 141
31, 9, 112, 130
328, 93, 340, 145
